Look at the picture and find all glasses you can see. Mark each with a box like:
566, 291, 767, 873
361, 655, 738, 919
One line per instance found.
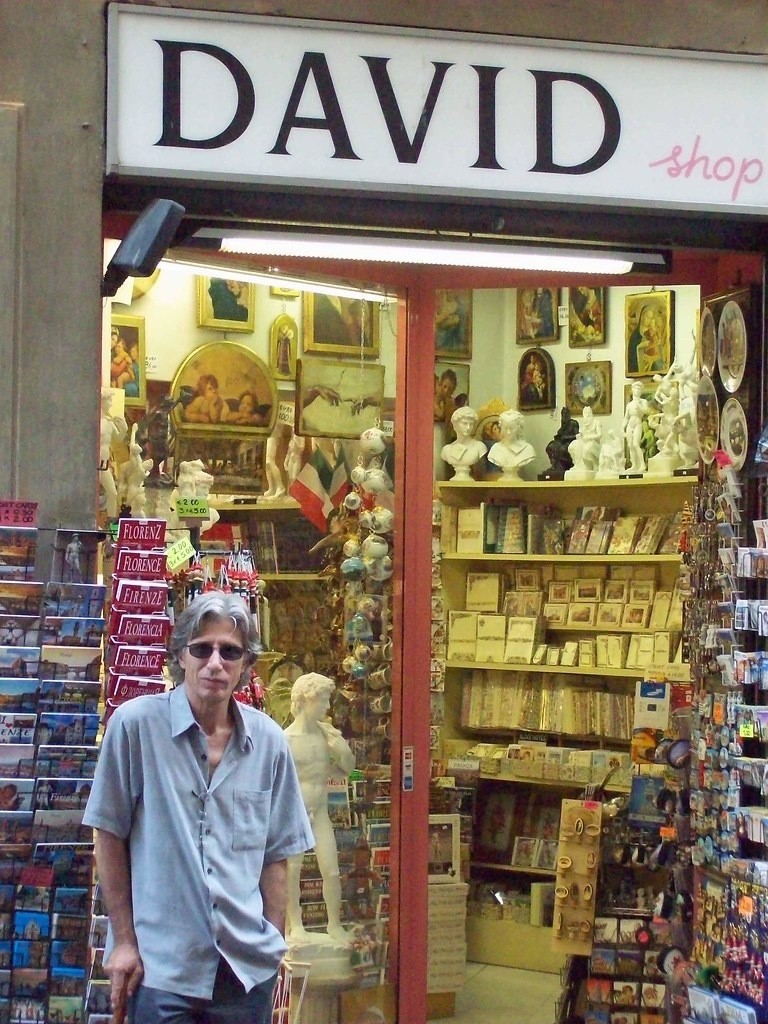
184, 644, 245, 661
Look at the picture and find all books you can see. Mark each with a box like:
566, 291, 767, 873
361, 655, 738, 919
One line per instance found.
441, 502, 683, 669
0, 526, 117, 1024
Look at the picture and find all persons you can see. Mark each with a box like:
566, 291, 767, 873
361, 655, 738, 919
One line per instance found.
263, 424, 305, 498
545, 407, 580, 470
0, 783, 17, 810
568, 406, 602, 469
83, 591, 315, 1024
65, 533, 85, 584
486, 409, 536, 468
37, 780, 53, 810
647, 330, 696, 467
98, 387, 220, 533
441, 407, 488, 467
281, 674, 358, 943
621, 381, 651, 471
600, 429, 622, 470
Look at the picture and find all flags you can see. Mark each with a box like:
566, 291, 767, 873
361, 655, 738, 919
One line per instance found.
289, 449, 336, 533
330, 448, 349, 508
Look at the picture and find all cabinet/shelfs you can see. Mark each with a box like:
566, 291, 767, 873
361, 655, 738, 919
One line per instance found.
434, 475, 702, 978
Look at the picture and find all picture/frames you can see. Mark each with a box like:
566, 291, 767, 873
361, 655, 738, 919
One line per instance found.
101, 242, 394, 444
434, 285, 677, 480
